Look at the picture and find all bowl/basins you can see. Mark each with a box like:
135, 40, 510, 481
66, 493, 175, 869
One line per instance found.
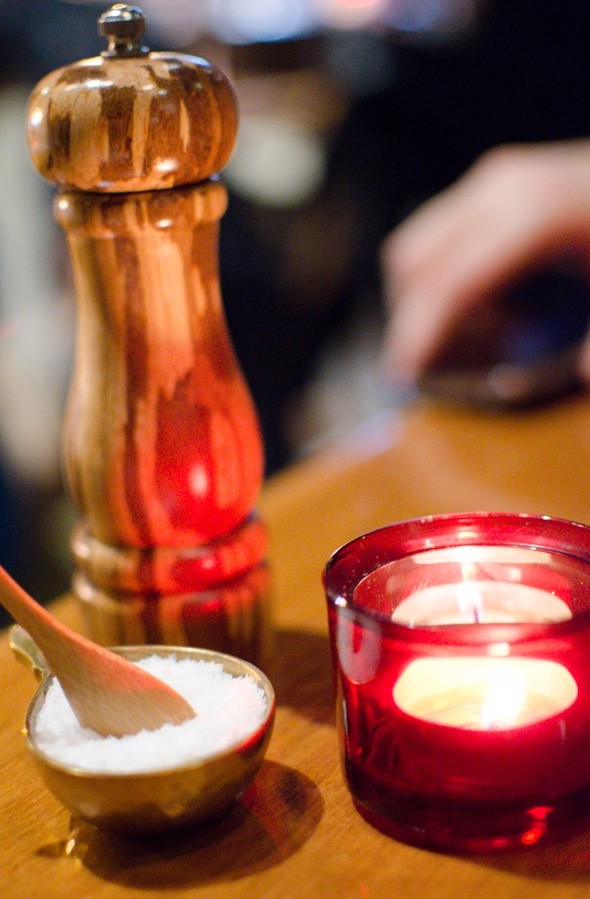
10, 619, 276, 836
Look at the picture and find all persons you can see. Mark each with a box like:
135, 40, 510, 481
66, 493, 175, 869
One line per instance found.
379, 139, 590, 407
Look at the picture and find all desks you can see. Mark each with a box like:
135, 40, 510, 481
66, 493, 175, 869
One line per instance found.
0, 388, 590, 899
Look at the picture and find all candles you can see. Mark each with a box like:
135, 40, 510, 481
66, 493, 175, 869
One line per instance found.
387, 565, 579, 730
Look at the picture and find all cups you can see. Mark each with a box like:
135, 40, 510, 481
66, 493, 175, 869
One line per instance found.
320, 512, 590, 853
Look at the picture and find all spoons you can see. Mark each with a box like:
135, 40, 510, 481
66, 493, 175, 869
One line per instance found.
1, 569, 196, 738
